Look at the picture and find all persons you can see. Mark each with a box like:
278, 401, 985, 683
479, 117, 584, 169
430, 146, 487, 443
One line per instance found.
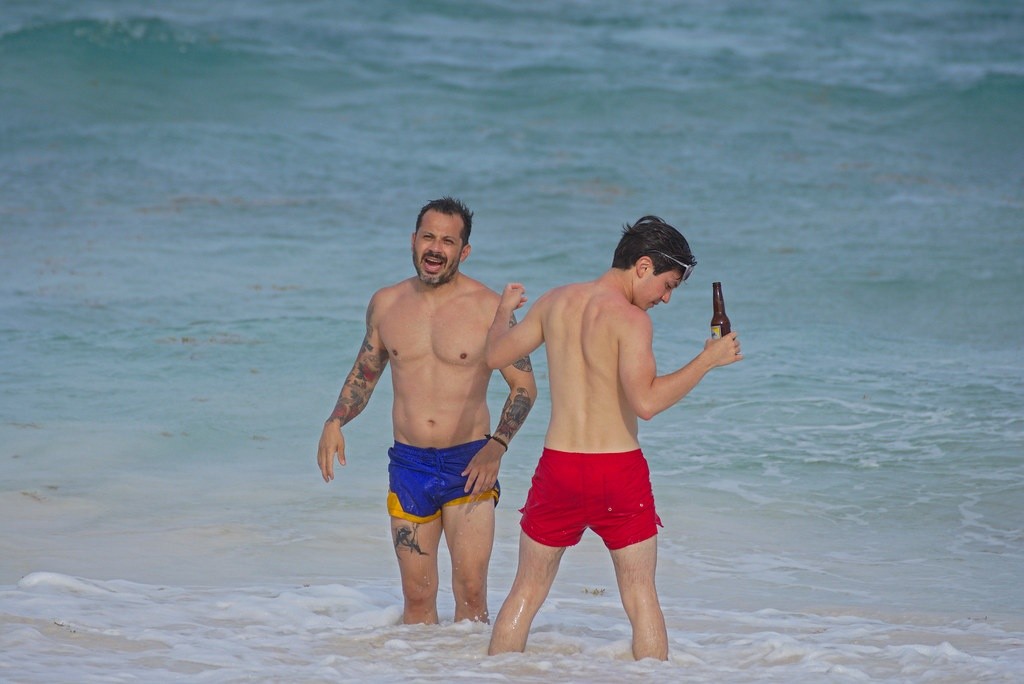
316, 195, 537, 625
485, 215, 744, 661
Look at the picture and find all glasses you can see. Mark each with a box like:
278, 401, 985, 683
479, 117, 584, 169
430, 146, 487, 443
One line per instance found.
646, 249, 694, 281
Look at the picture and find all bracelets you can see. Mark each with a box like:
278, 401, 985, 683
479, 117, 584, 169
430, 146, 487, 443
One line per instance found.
484, 434, 508, 452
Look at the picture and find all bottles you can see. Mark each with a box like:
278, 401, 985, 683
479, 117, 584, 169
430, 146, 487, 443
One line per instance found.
711, 282, 731, 339
